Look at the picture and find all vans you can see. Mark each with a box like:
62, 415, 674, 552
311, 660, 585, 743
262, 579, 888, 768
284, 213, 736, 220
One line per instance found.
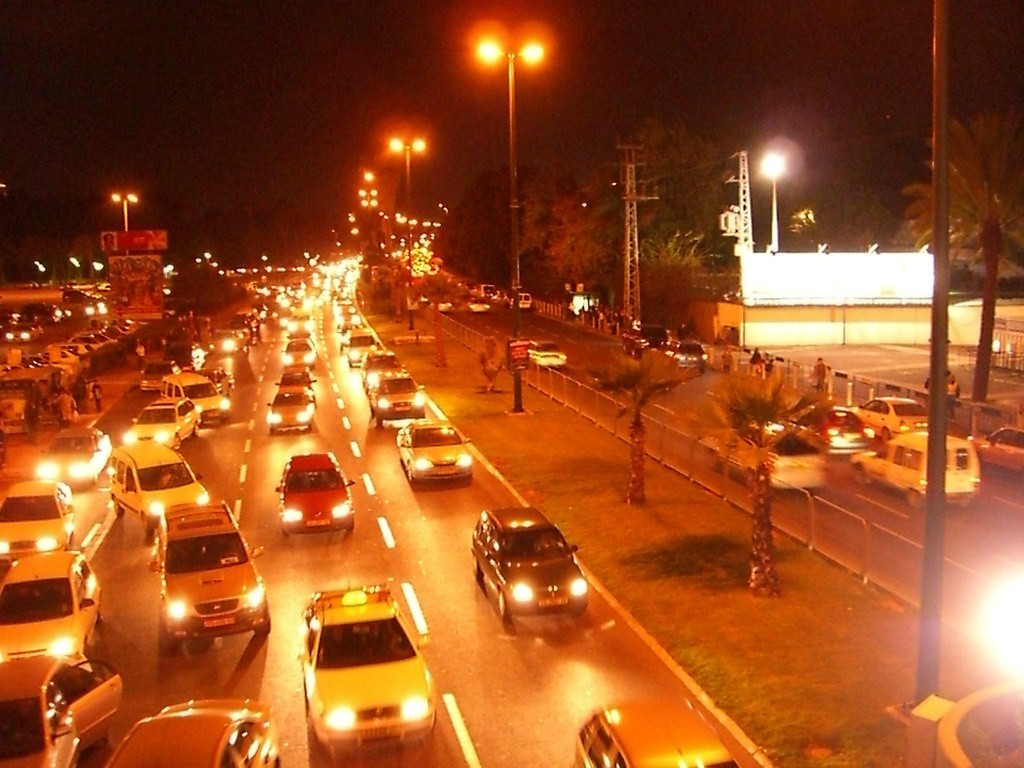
108, 441, 210, 536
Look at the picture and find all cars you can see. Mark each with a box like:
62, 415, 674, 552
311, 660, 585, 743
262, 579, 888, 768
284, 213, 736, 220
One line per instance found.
0, 479, 75, 561
395, 420, 473, 486
275, 450, 358, 538
34, 425, 113, 486
296, 582, 441, 756
703, 419, 829, 497
0, 652, 125, 768
849, 396, 930, 441
0, 549, 103, 663
118, 395, 201, 451
786, 405, 877, 458
674, 343, 708, 374
637, 323, 671, 350
0, 282, 568, 439
102, 696, 282, 768
964, 423, 1024, 474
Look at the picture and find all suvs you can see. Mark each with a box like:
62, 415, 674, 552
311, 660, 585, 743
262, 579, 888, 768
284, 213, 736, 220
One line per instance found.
471, 505, 589, 624
571, 700, 740, 767
849, 429, 982, 512
146, 500, 272, 655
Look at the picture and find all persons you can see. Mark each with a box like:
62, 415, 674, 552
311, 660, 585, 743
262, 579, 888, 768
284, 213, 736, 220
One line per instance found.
47, 667, 109, 751
295, 472, 314, 492
948, 371, 960, 426
92, 380, 103, 412
103, 234, 115, 251
721, 348, 732, 374
153, 467, 181, 488
317, 471, 336, 489
133, 306, 209, 354
587, 304, 622, 336
40, 376, 93, 426
811, 357, 831, 396
196, 387, 215, 396
360, 624, 397, 659
487, 289, 510, 304
749, 346, 774, 382
135, 343, 145, 369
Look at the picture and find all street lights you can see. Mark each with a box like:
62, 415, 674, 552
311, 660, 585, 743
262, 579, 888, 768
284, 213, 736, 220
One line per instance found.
359, 189, 378, 227
475, 34, 545, 414
761, 152, 786, 252
389, 136, 427, 331
112, 193, 139, 256
